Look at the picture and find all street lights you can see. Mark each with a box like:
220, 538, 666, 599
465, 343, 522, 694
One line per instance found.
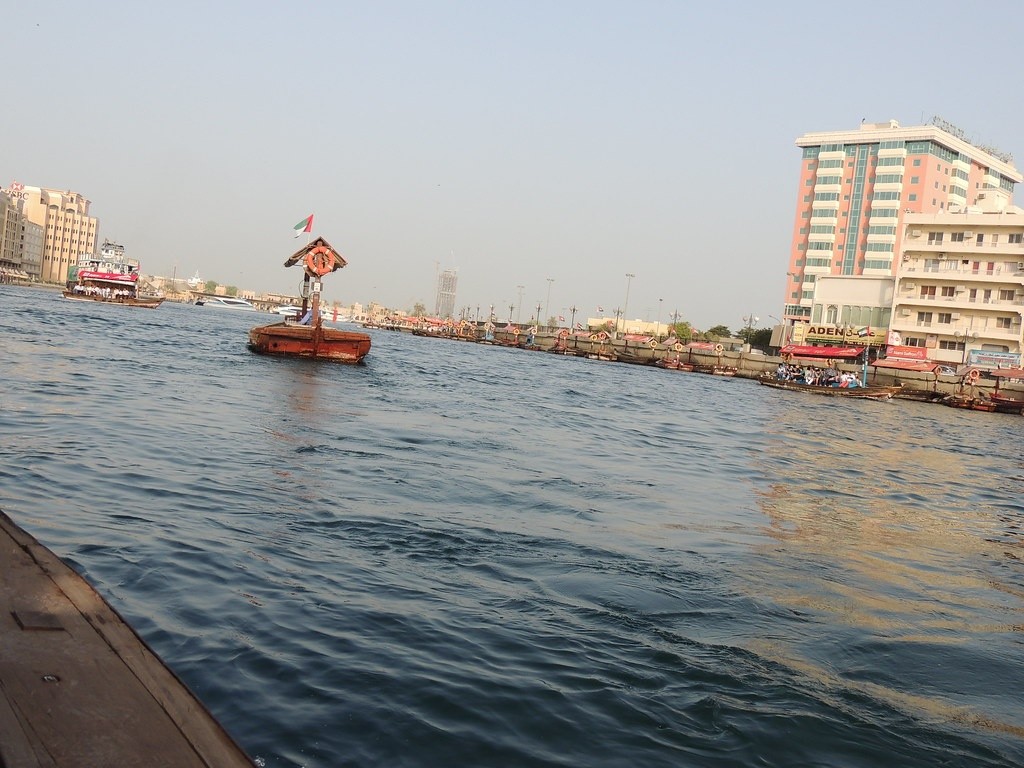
545, 278, 555, 324
570, 306, 579, 328
623, 272, 635, 333
955, 328, 980, 364
536, 305, 543, 324
509, 304, 515, 320
743, 314, 760, 343
669, 311, 682, 334
613, 307, 623, 330
657, 298, 664, 333
517, 285, 524, 321
835, 321, 855, 348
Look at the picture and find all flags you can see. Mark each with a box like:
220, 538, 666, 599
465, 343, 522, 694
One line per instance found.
672, 328, 676, 335
559, 316, 566, 322
577, 322, 583, 329
857, 326, 869, 338
293, 215, 313, 238
692, 328, 699, 336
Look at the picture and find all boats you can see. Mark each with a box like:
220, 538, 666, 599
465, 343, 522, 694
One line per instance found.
365, 317, 737, 379
755, 344, 902, 402
63, 271, 166, 309
249, 215, 372, 365
942, 374, 1023, 412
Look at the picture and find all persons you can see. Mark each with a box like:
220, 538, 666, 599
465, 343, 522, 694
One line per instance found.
73, 283, 136, 299
777, 363, 859, 388
